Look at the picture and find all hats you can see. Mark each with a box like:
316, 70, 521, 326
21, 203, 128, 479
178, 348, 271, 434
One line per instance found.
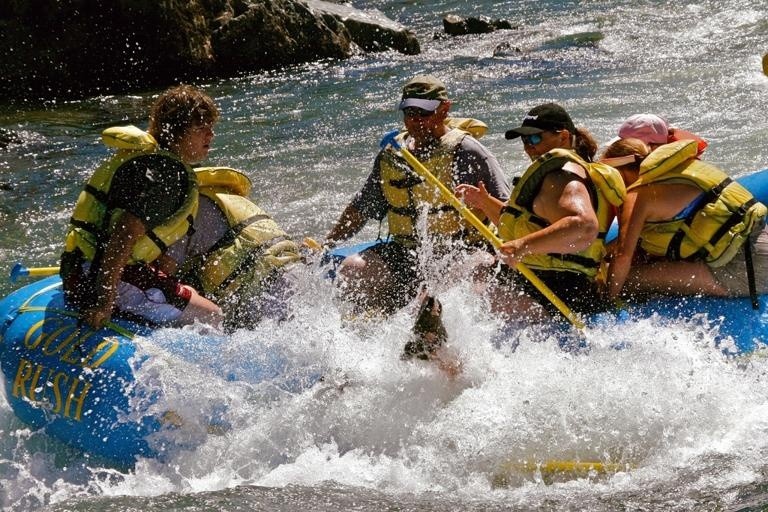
604, 112, 669, 148
504, 102, 576, 141
397, 73, 449, 112
597, 144, 639, 167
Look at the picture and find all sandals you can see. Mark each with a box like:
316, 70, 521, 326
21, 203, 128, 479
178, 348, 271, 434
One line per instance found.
397, 294, 449, 362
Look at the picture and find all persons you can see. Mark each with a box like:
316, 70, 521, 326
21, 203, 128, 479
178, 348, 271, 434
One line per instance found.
320, 72, 510, 329
400, 295, 461, 382
455, 102, 624, 327
164, 163, 307, 328
600, 136, 768, 301
618, 113, 671, 153
59, 85, 228, 332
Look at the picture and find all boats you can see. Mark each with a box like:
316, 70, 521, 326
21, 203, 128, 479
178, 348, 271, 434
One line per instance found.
0, 262, 372, 461
324, 164, 768, 355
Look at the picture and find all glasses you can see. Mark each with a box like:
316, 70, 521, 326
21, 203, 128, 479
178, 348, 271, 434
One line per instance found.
403, 107, 439, 116
521, 129, 563, 146
